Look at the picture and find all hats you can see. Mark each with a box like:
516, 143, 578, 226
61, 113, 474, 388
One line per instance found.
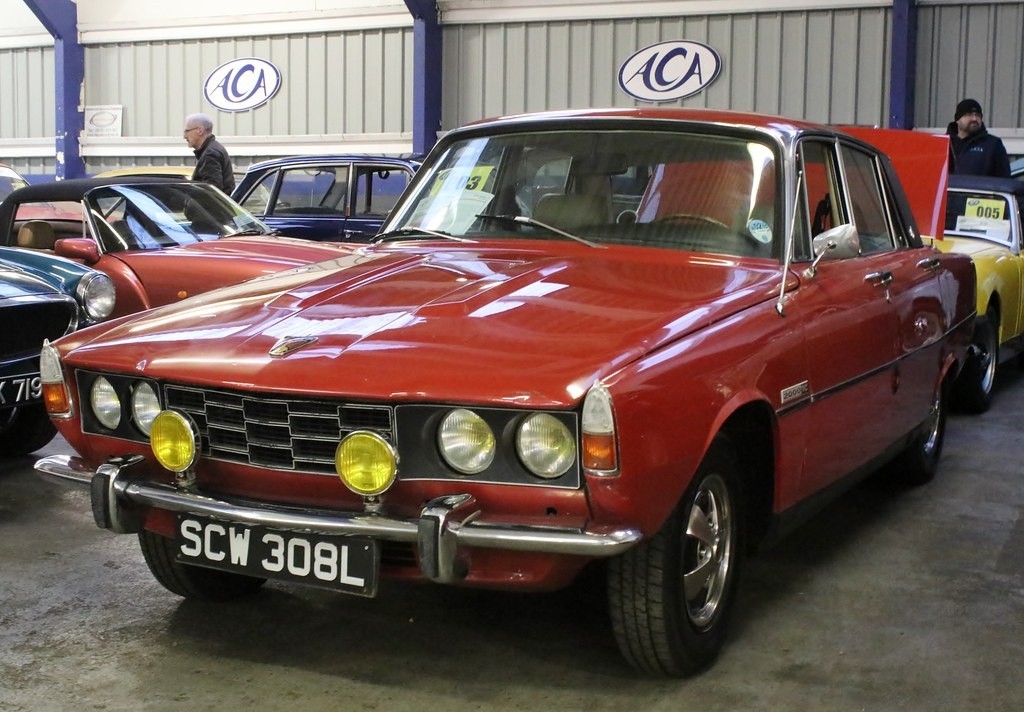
955, 99, 983, 121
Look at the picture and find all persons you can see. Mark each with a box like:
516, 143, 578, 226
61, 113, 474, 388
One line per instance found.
946, 98, 1012, 231
184, 113, 236, 234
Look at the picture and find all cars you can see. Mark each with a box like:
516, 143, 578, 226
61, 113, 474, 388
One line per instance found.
945, 174, 1024, 406
0, 163, 280, 250
1, 247, 117, 455
33, 107, 979, 680
227, 152, 425, 246
1, 177, 358, 335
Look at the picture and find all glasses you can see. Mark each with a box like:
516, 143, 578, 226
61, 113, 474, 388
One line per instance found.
963, 112, 982, 119
184, 127, 203, 135
